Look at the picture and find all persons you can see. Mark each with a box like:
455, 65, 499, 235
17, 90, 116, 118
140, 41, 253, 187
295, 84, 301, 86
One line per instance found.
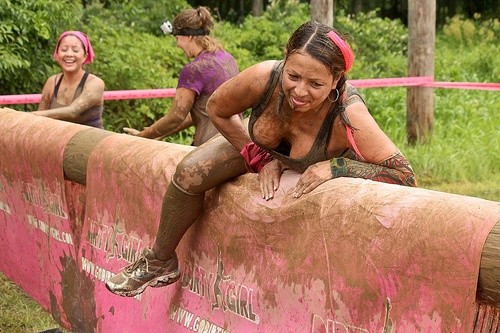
102, 21, 418, 297
30, 30, 104, 129
120, 6, 245, 148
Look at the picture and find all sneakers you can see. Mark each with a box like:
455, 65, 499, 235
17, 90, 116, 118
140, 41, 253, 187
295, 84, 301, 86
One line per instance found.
106, 246, 181, 297
